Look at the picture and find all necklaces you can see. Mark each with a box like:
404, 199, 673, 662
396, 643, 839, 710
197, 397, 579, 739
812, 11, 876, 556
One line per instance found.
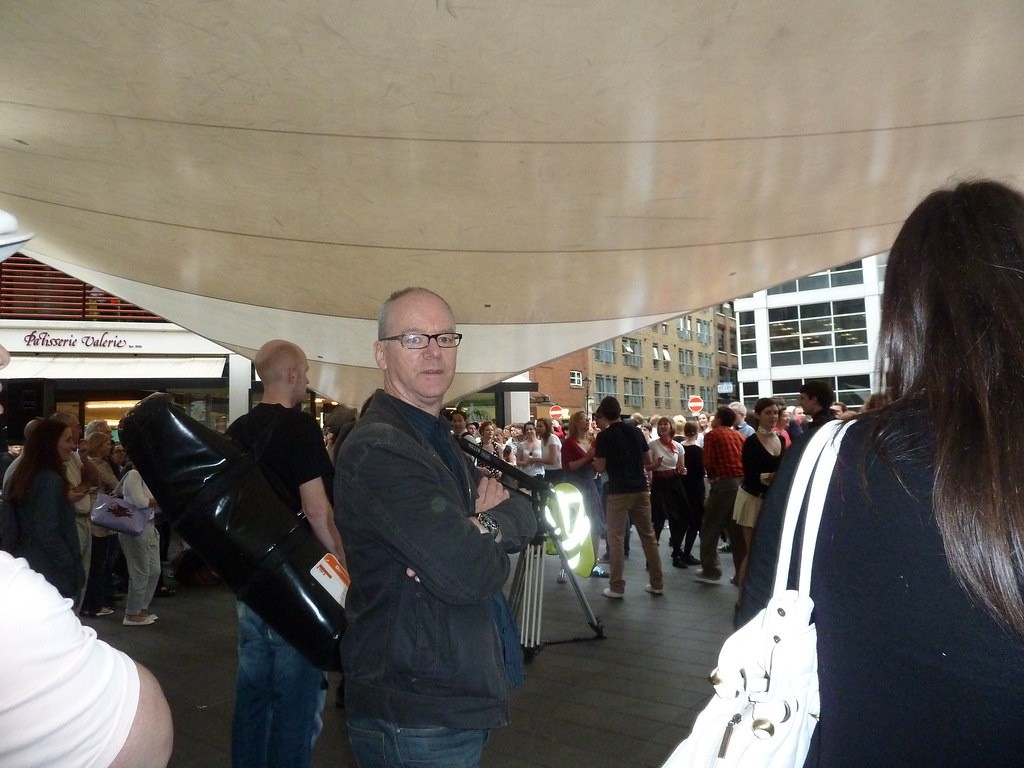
758, 425, 780, 437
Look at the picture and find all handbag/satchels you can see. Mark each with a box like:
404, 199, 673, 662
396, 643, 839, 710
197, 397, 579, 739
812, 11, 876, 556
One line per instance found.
89, 470, 154, 537
665, 421, 853, 768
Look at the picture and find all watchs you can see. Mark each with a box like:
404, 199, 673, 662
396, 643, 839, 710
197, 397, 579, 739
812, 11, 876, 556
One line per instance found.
474, 511, 499, 540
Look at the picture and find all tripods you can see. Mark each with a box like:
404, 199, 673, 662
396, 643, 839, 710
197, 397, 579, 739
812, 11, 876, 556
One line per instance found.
507, 482, 607, 663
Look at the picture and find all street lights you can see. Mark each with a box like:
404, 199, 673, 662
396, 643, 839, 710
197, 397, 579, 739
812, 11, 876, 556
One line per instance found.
582, 376, 592, 416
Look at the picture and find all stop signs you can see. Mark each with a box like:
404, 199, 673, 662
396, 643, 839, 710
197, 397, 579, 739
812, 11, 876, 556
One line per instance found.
549, 405, 563, 419
688, 396, 704, 412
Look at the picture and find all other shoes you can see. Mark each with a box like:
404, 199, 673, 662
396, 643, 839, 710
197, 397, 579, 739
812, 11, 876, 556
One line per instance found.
603, 552, 628, 562
590, 563, 610, 577
603, 589, 624, 598
729, 576, 739, 586
122, 612, 160, 626
672, 553, 688, 570
558, 573, 568, 584
154, 585, 177, 598
717, 543, 733, 554
645, 585, 663, 594
695, 568, 723, 582
83, 605, 116, 616
686, 554, 702, 566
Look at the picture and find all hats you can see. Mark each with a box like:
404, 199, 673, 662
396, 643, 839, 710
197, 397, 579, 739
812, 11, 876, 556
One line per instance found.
82, 432, 110, 455
325, 405, 357, 437
466, 421, 479, 430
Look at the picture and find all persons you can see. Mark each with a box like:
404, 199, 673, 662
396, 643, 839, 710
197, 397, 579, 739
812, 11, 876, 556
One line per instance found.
557, 410, 610, 583
736, 182, 1024, 768
694, 406, 746, 586
449, 392, 891, 569
322, 396, 372, 463
122, 469, 161, 625
0, 413, 176, 617
224, 339, 346, 768
0, 550, 174, 768
799, 381, 838, 428
734, 398, 786, 613
592, 396, 664, 598
332, 287, 537, 768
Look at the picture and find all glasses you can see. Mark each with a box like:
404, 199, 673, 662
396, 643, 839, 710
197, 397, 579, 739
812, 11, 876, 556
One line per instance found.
575, 417, 591, 422
380, 333, 462, 349
112, 449, 125, 454
